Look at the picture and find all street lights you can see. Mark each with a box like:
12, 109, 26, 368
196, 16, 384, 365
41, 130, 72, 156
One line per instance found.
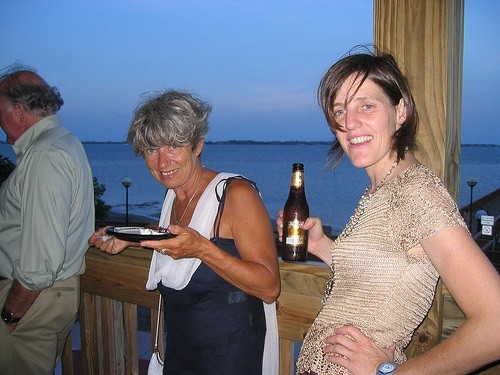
466, 178, 478, 233
121, 177, 132, 227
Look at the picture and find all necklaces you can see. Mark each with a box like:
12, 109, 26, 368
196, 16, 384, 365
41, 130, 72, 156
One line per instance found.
345, 146, 408, 237
173, 165, 205, 225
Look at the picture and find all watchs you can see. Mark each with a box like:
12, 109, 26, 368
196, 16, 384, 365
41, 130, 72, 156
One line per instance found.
1, 307, 22, 324
377, 361, 398, 375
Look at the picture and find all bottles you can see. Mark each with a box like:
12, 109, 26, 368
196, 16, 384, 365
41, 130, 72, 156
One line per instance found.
282, 162, 308, 261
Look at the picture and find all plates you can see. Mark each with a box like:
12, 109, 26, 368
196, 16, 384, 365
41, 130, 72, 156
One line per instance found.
106, 226, 172, 239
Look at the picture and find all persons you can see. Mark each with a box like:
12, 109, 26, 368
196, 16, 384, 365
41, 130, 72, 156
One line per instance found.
275, 44, 500, 375
89, 90, 282, 375
0, 63, 94, 375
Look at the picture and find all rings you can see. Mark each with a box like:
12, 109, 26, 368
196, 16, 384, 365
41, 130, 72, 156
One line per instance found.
160, 248, 166, 256
